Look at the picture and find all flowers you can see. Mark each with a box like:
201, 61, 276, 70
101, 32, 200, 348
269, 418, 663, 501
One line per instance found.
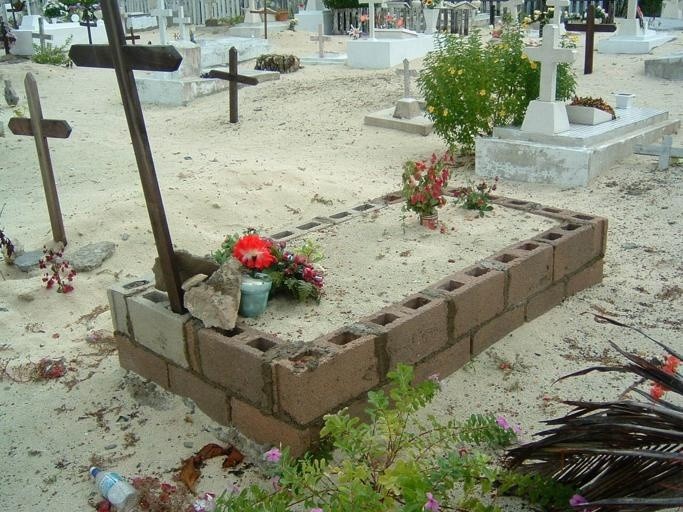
393, 152, 449, 234
569, 93, 616, 118
234, 233, 275, 279
420, 0, 441, 8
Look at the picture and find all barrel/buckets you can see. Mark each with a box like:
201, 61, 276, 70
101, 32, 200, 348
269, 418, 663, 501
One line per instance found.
420, 211, 438, 230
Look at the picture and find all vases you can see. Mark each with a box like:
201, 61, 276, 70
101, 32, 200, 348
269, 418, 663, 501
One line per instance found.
418, 212, 439, 230
237, 279, 272, 318
422, 8, 439, 34
276, 11, 289, 21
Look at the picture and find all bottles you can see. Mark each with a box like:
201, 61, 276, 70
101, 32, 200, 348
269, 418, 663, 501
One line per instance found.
90, 466, 136, 509
